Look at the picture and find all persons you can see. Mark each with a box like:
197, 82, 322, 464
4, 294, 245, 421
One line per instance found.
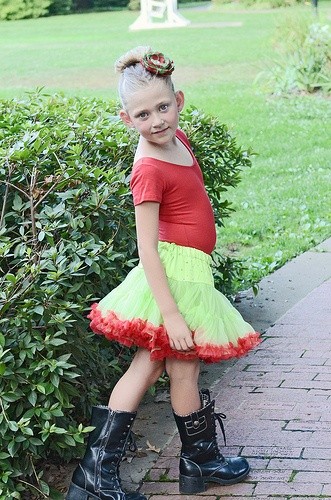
66, 44, 261, 500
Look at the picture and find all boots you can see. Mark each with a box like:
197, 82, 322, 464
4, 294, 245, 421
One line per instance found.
66, 405, 147, 500
173, 389, 250, 493
